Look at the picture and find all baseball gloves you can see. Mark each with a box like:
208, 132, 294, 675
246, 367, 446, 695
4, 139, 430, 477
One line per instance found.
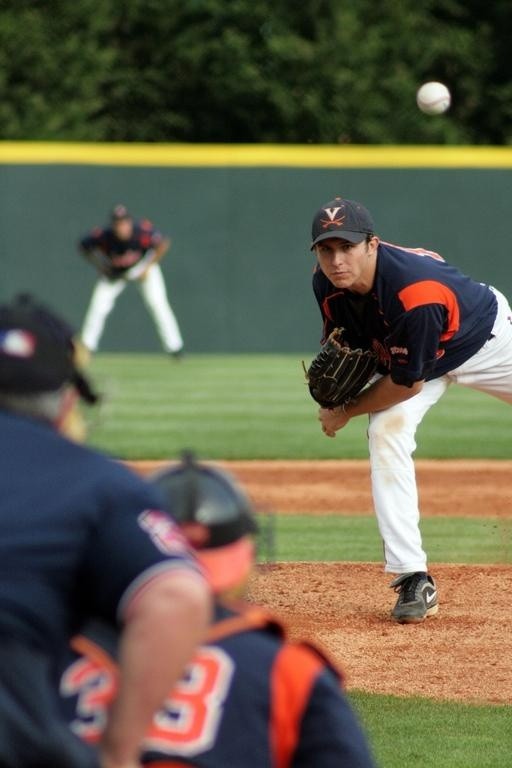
306, 327, 382, 410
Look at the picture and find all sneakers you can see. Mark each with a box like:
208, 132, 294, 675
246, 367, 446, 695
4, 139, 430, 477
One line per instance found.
393, 575, 438, 624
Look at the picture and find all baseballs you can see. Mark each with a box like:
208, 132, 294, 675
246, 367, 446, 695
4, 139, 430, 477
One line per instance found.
416, 77, 451, 116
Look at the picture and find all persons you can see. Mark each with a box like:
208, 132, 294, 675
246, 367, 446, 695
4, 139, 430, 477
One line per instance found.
54, 449, 372, 768
0, 288, 215, 768
308, 196, 512, 624
78, 204, 185, 363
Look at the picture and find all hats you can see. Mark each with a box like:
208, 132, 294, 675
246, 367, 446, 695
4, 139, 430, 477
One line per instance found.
151, 463, 261, 550
310, 198, 374, 251
0, 292, 98, 404
110, 203, 132, 221
